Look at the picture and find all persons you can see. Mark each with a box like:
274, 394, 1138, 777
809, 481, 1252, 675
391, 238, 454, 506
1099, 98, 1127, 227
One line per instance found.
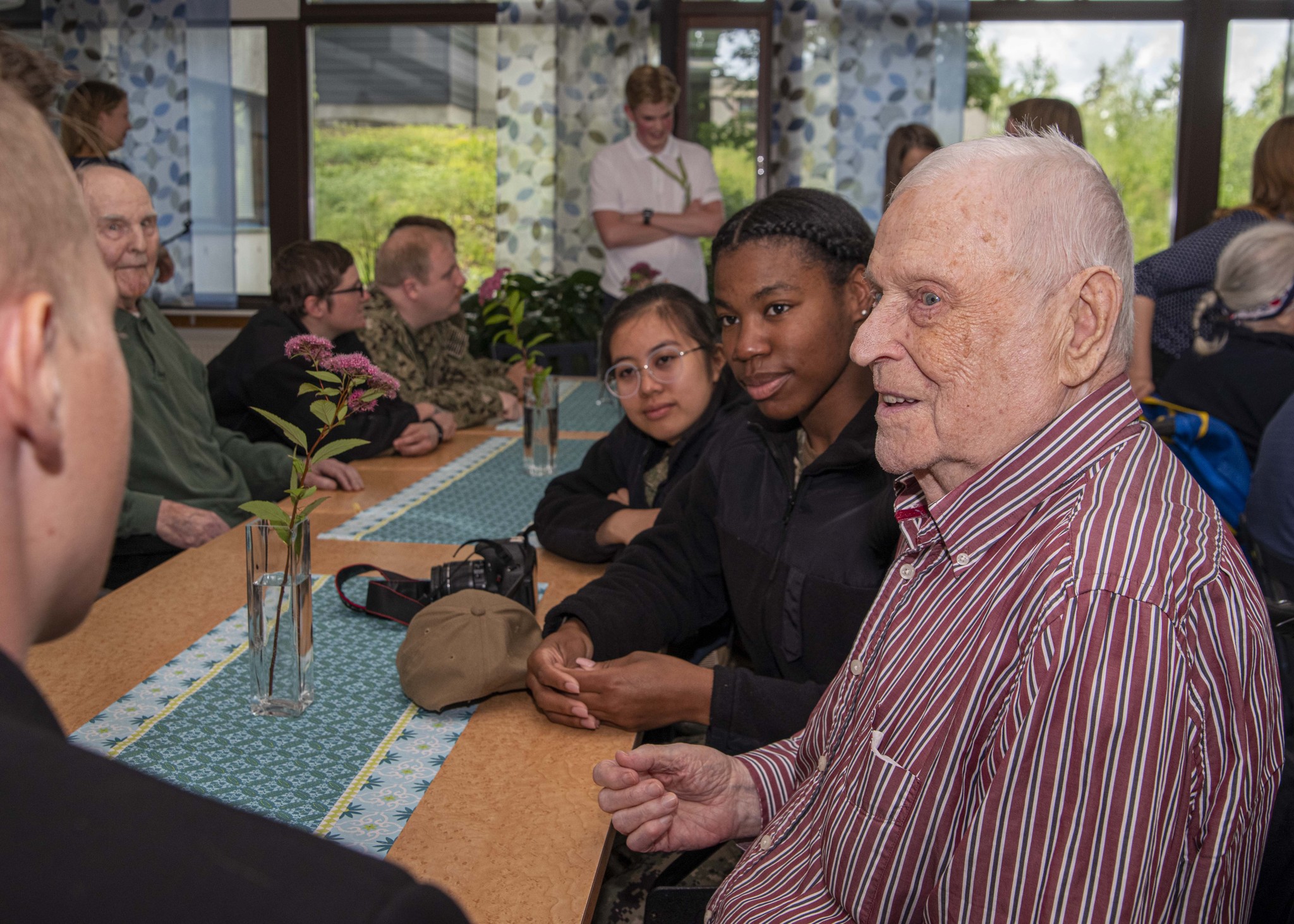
67, 155, 366, 602
532, 283, 728, 564
584, 135, 1283, 924
0, 37, 472, 924
52, 75, 177, 284
587, 63, 727, 378
200, 239, 459, 464
347, 215, 547, 429
876, 97, 1293, 650
528, 184, 903, 924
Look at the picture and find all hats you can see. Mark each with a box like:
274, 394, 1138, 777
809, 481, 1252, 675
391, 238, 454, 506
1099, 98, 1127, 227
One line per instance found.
395, 590, 544, 715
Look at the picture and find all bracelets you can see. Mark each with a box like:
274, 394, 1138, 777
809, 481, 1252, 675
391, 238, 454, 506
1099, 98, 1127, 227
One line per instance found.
643, 209, 653, 225
422, 418, 444, 445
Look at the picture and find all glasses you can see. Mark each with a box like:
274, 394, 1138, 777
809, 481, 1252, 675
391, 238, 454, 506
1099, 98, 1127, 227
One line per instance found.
604, 346, 704, 399
326, 282, 366, 299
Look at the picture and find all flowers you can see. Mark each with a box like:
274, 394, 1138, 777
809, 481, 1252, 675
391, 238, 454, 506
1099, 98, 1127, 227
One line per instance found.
239, 333, 403, 696
477, 264, 553, 421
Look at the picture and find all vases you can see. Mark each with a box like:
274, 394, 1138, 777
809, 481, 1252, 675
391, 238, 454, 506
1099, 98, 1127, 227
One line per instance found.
518, 377, 558, 478
245, 521, 310, 720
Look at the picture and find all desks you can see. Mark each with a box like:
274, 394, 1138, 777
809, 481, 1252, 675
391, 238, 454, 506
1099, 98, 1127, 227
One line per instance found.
24, 376, 645, 924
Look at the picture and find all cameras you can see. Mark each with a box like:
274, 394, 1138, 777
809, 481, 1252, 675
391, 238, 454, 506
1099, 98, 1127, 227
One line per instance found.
428, 540, 536, 613
